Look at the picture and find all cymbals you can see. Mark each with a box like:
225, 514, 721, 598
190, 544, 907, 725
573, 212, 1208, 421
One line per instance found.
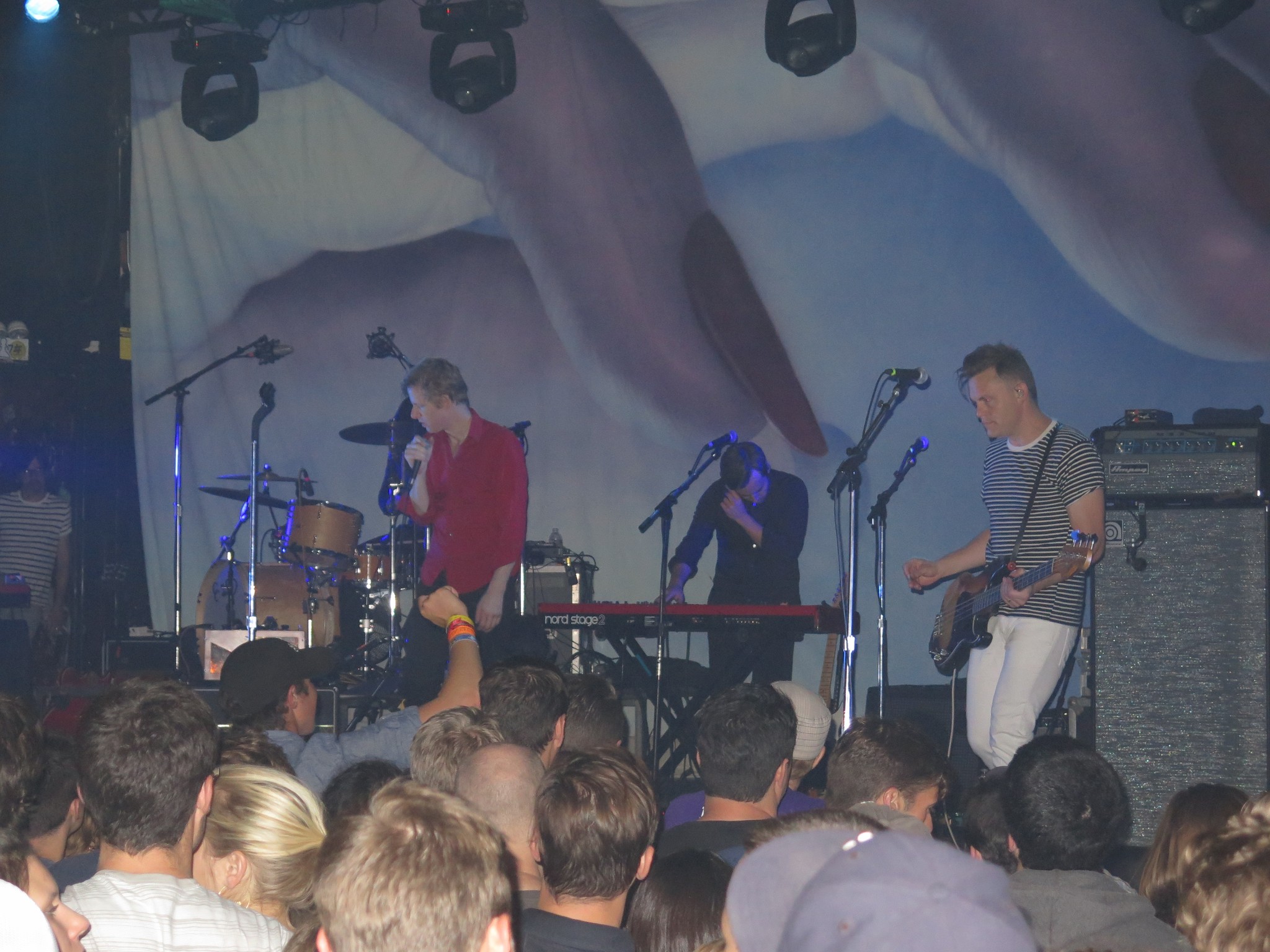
339, 419, 425, 448
215, 471, 319, 483
197, 485, 290, 511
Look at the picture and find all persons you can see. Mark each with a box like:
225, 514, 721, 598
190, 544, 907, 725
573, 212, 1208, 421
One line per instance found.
902, 341, 1107, 773
650, 440, 810, 699
394, 356, 531, 710
0, 446, 76, 710
0, 582, 1270, 952
342, 396, 427, 649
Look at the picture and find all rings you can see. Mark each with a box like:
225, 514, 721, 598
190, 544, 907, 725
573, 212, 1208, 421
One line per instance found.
54, 627, 58, 630
1007, 599, 1011, 602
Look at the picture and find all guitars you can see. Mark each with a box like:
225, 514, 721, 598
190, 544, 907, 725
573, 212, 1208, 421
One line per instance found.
927, 528, 1097, 679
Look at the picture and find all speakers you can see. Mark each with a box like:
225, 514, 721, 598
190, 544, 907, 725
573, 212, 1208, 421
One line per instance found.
1090, 427, 1270, 849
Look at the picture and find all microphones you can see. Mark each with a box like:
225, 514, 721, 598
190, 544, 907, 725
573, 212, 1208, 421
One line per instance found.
408, 439, 429, 486
702, 431, 738, 450
884, 366, 929, 385
241, 344, 294, 359
906, 436, 929, 455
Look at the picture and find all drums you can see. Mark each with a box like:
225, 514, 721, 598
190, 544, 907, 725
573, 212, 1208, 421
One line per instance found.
194, 557, 335, 672
333, 549, 398, 586
277, 499, 364, 571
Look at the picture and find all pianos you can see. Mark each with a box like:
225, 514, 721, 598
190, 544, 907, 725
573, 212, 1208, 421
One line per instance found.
537, 600, 863, 637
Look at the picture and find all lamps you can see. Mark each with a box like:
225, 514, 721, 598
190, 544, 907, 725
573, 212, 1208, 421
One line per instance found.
415, 1, 528, 114
172, 3, 272, 141
764, 0, 855, 81
